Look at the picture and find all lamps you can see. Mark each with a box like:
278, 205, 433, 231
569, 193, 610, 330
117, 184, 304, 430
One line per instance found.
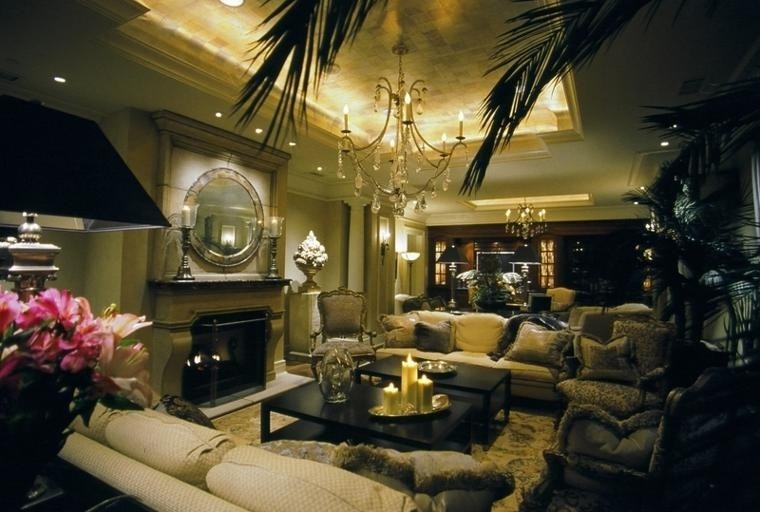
171, 199, 204, 280
261, 213, 288, 281
499, 197, 553, 242
436, 244, 469, 306
400, 251, 421, 293
0, 91, 167, 294
334, 69, 475, 218
379, 225, 394, 260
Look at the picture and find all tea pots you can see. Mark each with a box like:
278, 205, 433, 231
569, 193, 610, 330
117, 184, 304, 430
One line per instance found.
309, 345, 358, 405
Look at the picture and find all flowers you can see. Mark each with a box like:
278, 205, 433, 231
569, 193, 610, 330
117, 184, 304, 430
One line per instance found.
1, 281, 152, 421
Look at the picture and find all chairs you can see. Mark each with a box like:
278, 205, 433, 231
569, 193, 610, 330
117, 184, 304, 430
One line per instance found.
549, 309, 679, 426
516, 361, 737, 512
310, 286, 377, 384
526, 287, 553, 317
544, 282, 577, 312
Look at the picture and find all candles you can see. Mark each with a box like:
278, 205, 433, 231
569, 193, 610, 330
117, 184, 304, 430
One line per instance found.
397, 356, 417, 409
416, 375, 433, 412
382, 380, 400, 416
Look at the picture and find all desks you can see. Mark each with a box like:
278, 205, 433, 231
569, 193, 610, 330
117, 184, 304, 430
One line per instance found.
14, 456, 69, 511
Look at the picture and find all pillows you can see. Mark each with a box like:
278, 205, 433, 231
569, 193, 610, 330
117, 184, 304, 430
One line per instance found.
374, 309, 429, 350
323, 437, 515, 510
151, 389, 216, 433
548, 398, 665, 475
502, 317, 576, 372
249, 433, 351, 474
572, 329, 642, 384
412, 318, 456, 355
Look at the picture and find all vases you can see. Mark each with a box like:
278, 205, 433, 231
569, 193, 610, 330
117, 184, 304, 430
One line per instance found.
0, 375, 49, 512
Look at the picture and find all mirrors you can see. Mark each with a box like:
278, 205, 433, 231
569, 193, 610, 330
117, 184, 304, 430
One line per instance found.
182, 164, 266, 268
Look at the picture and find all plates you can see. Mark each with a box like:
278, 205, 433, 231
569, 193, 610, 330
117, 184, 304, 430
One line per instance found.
417, 358, 460, 379
366, 399, 454, 419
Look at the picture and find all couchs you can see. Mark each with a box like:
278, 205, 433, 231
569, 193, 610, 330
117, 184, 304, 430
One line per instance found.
378, 304, 582, 407
56, 372, 517, 511
